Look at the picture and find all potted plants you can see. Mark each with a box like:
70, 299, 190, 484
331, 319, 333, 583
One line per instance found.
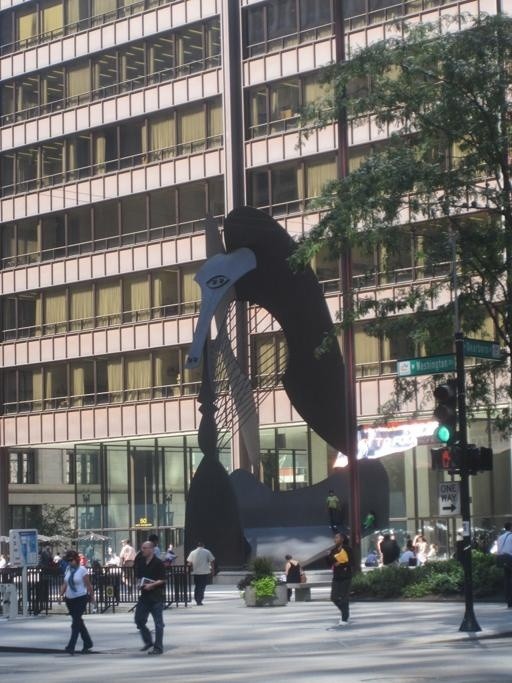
245, 576, 287, 607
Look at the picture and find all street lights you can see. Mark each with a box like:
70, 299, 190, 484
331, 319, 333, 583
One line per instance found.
163, 492, 174, 527
81, 487, 92, 531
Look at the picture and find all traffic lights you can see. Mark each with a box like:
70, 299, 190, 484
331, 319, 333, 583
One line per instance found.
430, 379, 460, 443
461, 442, 495, 474
430, 446, 460, 470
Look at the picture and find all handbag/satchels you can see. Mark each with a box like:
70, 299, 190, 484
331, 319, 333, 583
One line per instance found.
300, 571, 307, 583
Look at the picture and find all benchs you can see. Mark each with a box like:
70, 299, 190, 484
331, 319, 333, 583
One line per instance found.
285, 581, 332, 602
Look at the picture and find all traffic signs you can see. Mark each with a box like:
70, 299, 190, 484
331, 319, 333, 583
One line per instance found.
462, 334, 504, 362
393, 353, 460, 377
437, 480, 462, 515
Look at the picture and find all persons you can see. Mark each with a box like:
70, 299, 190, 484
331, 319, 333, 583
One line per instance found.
56, 550, 98, 656
326, 489, 342, 529
282, 554, 301, 602
497, 521, 512, 608
133, 540, 166, 656
327, 531, 353, 626
360, 511, 376, 529
0, 533, 216, 616
364, 527, 439, 568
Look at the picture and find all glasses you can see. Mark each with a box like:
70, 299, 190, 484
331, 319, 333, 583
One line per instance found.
68, 558, 73, 563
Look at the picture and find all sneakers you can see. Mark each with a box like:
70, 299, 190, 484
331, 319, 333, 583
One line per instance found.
62, 645, 76, 655
81, 641, 93, 653
138, 640, 153, 651
147, 647, 164, 654
193, 596, 204, 606
